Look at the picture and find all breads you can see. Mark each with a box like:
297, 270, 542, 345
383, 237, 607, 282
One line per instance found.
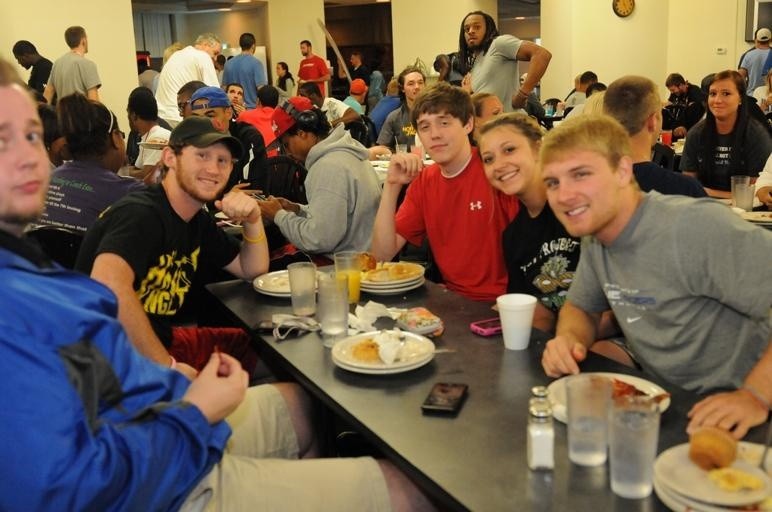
352, 250, 376, 272
589, 375, 670, 409
397, 305, 441, 330
690, 424, 737, 469
354, 337, 382, 365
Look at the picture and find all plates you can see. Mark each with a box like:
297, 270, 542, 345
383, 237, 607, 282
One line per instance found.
364, 142, 436, 171
136, 141, 169, 150
550, 371, 670, 425
252, 260, 435, 376
709, 190, 772, 226
651, 439, 772, 512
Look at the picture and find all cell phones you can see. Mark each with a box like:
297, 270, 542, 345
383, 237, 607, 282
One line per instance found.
421, 382, 469, 416
252, 320, 299, 336
470, 317, 501, 337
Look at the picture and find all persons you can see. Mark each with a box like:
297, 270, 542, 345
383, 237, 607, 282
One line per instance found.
4, 10, 772, 511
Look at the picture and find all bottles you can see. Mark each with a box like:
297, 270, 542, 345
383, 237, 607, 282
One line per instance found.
526, 386, 556, 473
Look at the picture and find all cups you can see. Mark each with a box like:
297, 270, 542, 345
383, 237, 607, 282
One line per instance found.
524, 465, 654, 512
335, 250, 362, 304
656, 130, 685, 155
564, 373, 612, 465
730, 174, 755, 212
496, 292, 539, 348
544, 103, 564, 117
498, 349, 535, 399
607, 399, 661, 499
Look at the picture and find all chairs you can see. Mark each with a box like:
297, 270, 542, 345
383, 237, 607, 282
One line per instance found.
650, 143, 676, 172
264, 156, 305, 198
361, 114, 378, 143
666, 103, 686, 119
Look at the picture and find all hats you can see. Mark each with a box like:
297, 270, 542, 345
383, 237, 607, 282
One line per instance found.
168, 117, 247, 156
186, 87, 232, 114
755, 28, 772, 45
259, 98, 317, 152
349, 77, 368, 96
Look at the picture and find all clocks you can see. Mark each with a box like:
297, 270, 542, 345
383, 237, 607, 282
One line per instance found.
612, 0, 634, 17
